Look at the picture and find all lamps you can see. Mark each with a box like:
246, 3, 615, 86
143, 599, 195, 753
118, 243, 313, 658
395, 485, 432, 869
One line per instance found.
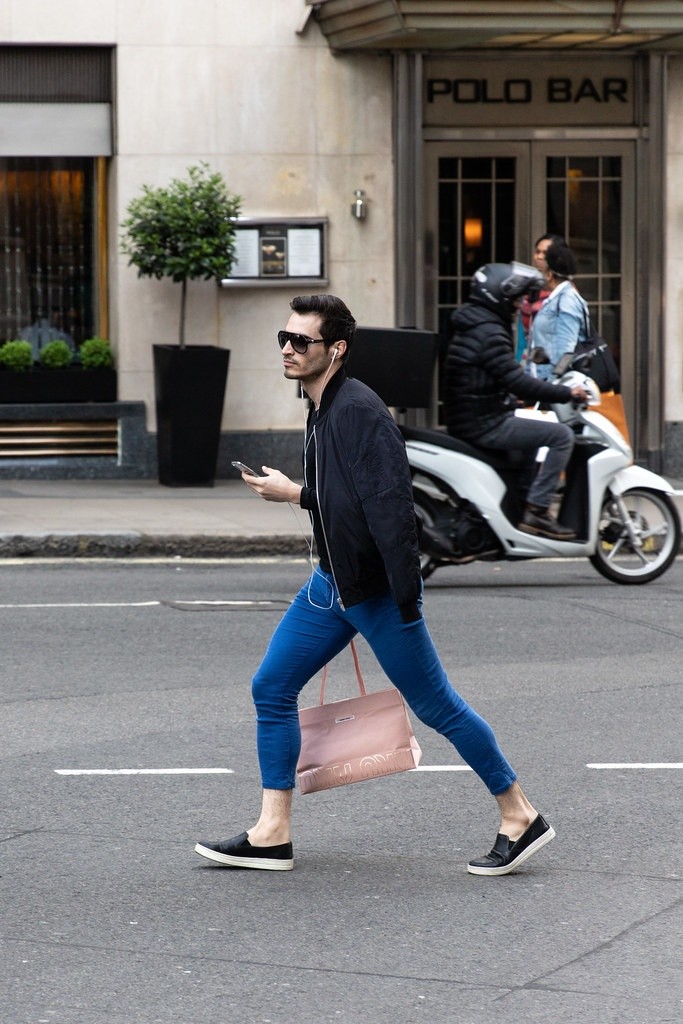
463, 217, 482, 248
351, 190, 368, 220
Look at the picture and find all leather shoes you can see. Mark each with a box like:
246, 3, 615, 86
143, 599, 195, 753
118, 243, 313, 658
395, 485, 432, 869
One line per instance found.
518, 506, 576, 539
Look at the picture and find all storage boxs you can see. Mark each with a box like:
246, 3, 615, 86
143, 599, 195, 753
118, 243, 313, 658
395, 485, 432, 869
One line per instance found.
297, 328, 442, 408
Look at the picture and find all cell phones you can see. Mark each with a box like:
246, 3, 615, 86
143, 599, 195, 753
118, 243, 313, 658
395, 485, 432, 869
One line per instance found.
231, 461, 260, 477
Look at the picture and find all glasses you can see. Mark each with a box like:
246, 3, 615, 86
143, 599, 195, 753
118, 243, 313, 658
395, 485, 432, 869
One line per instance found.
276, 331, 327, 354
518, 289, 541, 304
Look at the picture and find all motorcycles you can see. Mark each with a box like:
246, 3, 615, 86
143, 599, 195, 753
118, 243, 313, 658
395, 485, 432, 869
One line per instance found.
390, 341, 683, 586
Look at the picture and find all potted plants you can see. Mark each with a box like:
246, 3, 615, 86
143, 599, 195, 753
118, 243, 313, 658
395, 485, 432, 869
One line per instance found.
118, 162, 244, 486
0, 335, 116, 403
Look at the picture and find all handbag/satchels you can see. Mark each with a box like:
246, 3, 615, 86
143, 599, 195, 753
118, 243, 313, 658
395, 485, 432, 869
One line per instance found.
295, 687, 419, 794
556, 293, 619, 393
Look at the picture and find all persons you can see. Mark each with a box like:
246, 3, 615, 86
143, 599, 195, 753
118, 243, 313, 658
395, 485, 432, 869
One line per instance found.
439, 262, 588, 539
13, 304, 86, 362
195, 294, 556, 876
516, 234, 593, 500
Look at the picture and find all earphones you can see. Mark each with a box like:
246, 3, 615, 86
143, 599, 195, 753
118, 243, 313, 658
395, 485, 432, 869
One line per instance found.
331, 349, 338, 362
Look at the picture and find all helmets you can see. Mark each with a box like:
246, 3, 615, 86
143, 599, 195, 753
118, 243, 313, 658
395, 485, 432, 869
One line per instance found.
470, 264, 521, 321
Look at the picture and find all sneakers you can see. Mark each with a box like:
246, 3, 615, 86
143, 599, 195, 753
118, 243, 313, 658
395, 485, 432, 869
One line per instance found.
194, 832, 294, 871
466, 812, 556, 874
